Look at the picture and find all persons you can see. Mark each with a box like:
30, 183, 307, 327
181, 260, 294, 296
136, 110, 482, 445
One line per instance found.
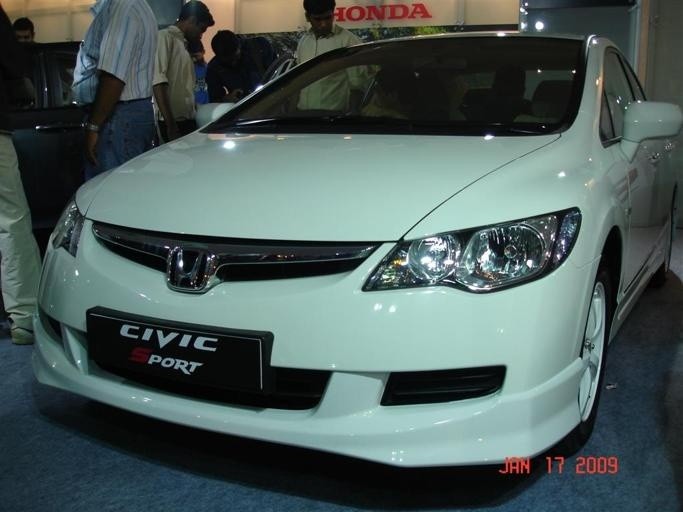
11, 16, 35, 43
70, 0, 156, 182
0, 7, 43, 346
204, 27, 275, 104
187, 40, 209, 105
289, 1, 365, 113
153, 0, 216, 147
369, 64, 419, 121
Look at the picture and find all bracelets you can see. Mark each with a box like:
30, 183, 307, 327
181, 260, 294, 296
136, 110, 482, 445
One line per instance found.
80, 119, 100, 132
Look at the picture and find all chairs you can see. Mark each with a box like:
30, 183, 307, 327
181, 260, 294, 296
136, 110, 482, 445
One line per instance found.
507, 78, 573, 125
360, 68, 422, 121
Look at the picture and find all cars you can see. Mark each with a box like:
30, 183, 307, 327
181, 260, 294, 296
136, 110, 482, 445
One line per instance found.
7, 40, 84, 265
31, 26, 680, 501
